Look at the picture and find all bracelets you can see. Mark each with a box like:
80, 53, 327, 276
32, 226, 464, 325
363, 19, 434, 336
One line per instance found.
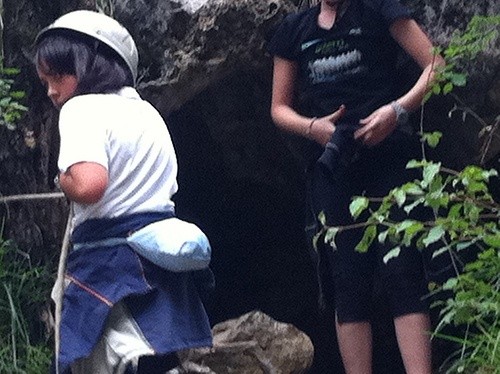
307, 117, 316, 140
54, 174, 62, 190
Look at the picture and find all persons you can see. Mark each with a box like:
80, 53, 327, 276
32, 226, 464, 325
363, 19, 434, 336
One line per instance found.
266, 0, 446, 374
22, 10, 213, 374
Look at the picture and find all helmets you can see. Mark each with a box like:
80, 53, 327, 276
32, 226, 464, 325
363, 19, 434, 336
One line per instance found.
35, 9, 140, 89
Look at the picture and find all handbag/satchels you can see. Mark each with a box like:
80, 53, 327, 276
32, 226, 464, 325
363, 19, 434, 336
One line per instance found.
74, 215, 211, 272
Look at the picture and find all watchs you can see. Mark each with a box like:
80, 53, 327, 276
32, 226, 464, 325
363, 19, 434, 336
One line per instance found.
391, 101, 409, 125
